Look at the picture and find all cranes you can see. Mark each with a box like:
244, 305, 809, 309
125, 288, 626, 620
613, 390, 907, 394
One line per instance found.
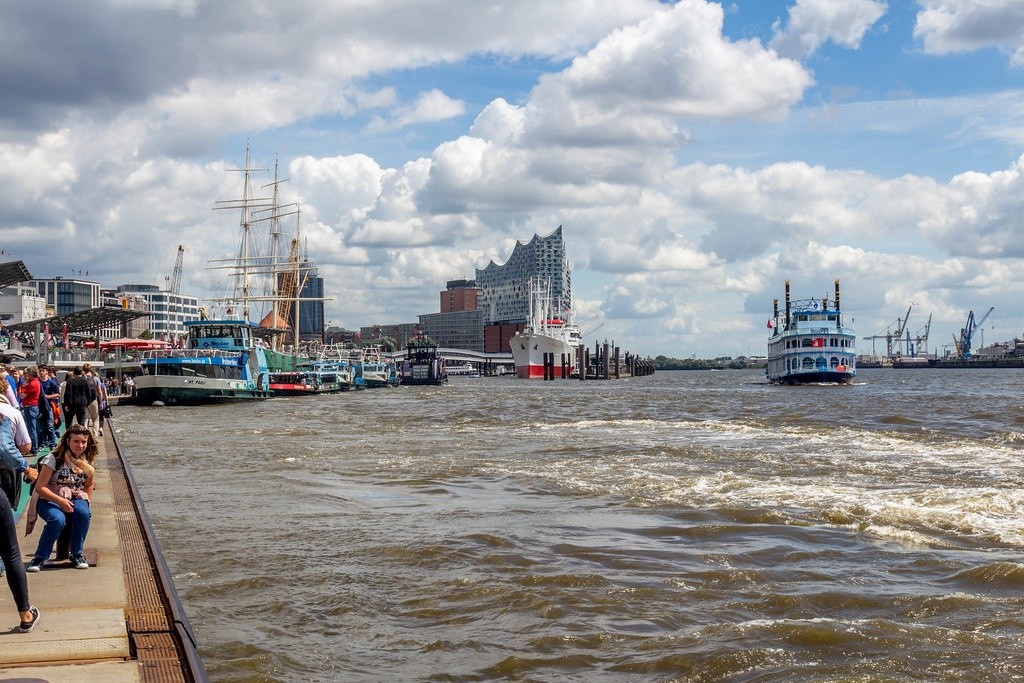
861, 307, 933, 362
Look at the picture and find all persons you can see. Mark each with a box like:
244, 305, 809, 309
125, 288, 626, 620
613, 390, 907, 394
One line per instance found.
26, 423, 99, 570
0, 363, 134, 631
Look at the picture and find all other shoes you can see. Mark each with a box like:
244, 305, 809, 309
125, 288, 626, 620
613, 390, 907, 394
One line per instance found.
99, 426, 103, 436
38, 447, 44, 451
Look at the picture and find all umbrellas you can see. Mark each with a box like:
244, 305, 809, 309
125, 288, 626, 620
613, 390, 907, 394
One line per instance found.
84, 336, 171, 352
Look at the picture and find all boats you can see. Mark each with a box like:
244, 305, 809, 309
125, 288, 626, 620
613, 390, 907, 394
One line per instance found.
765, 278, 858, 387
508, 274, 604, 379
398, 331, 449, 386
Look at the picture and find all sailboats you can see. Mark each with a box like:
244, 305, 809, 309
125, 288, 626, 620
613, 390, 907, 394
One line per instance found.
133, 136, 398, 405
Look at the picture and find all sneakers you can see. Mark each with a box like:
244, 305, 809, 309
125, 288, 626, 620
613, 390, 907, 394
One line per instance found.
70, 552, 88, 569
19, 605, 40, 633
27, 558, 45, 571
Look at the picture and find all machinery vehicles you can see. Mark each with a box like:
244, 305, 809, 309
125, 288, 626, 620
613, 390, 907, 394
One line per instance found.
951, 307, 995, 360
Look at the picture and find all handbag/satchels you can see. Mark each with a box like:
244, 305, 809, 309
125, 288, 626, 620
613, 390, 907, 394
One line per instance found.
105, 405, 112, 418
38, 384, 51, 412
